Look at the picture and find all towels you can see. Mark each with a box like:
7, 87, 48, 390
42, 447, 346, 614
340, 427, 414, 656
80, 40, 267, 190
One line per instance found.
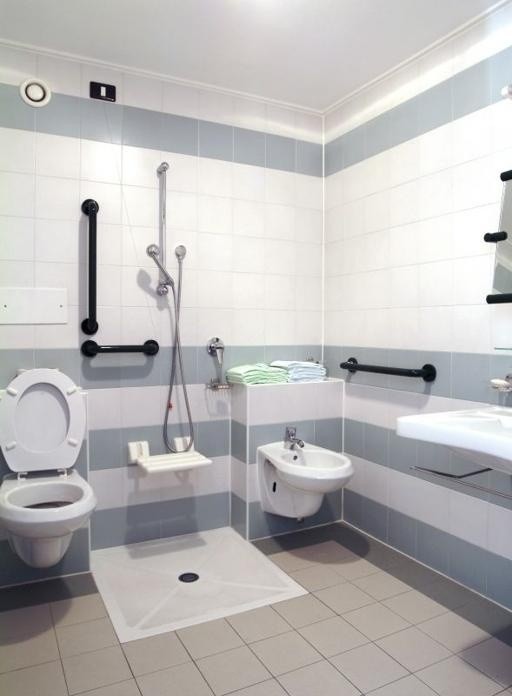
225, 358, 328, 387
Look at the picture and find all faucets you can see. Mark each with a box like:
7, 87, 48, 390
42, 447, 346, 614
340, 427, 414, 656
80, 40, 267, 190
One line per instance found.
285, 426, 305, 450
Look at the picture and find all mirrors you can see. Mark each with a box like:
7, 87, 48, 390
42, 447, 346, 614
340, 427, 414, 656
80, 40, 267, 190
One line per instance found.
485, 168, 512, 305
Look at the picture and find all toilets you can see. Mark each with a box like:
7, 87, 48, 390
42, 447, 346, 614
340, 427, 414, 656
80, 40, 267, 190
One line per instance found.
0, 368, 97, 569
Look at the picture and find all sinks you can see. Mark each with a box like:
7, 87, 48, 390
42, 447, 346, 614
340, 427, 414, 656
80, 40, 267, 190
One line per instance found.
254, 440, 355, 520
397, 404, 512, 462
